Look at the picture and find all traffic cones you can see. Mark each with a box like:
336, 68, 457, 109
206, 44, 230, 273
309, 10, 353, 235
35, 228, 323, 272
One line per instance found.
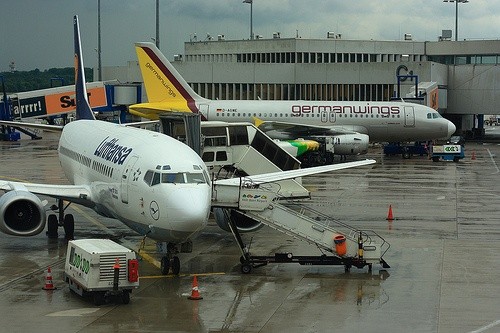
386, 203, 398, 220
42, 266, 57, 290
187, 275, 203, 300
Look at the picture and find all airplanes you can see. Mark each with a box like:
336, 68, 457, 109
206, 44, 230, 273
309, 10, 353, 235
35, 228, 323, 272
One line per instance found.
129, 40, 456, 163
0, 14, 391, 276
